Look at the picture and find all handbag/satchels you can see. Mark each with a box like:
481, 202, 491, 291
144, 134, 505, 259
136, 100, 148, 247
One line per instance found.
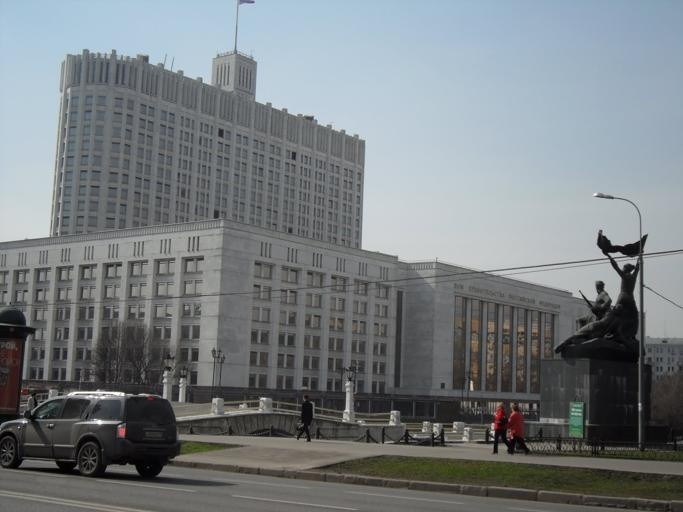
500, 416, 508, 426
296, 419, 305, 432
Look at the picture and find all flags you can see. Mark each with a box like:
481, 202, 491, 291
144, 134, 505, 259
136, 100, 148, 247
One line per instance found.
237, 0, 255, 6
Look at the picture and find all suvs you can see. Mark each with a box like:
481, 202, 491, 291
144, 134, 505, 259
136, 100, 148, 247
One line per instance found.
0, 391, 181, 477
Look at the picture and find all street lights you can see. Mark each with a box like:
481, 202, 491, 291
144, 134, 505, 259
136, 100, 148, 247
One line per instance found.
211, 347, 225, 399
343, 367, 355, 422
162, 354, 175, 401
179, 366, 187, 402
594, 192, 644, 452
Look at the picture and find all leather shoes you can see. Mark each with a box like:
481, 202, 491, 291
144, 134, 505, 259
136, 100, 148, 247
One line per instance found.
295, 435, 299, 440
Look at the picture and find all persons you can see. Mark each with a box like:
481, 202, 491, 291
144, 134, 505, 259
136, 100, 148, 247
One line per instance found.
491, 401, 512, 455
504, 400, 531, 457
575, 279, 613, 327
26, 392, 37, 410
588, 248, 642, 339
296, 394, 313, 442
